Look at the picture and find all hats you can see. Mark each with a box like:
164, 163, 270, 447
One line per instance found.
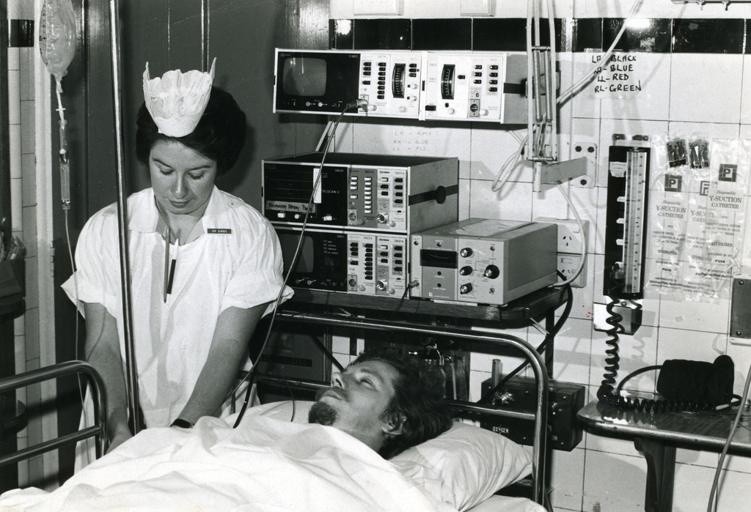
140, 57, 218, 139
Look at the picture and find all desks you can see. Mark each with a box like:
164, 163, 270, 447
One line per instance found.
576, 388, 751, 512
239, 281, 574, 512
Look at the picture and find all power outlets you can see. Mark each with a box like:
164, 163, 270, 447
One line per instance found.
558, 217, 590, 254
553, 254, 590, 289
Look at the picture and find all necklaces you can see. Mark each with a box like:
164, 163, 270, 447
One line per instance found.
153, 198, 207, 246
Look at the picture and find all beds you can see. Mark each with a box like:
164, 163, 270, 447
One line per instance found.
0, 305, 556, 512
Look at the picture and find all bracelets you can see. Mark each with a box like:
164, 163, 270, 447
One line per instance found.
169, 417, 194, 432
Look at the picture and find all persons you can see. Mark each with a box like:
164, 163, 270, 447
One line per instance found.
304, 347, 455, 467
60, 81, 296, 480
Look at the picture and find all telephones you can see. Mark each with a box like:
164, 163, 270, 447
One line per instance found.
657, 356, 735, 409
